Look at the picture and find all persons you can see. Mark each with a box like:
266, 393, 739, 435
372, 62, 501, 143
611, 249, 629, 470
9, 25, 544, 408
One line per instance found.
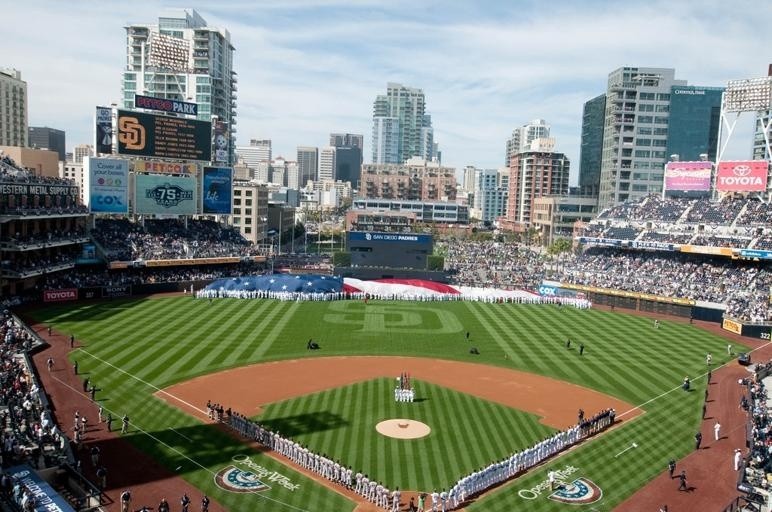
180, 492, 191, 512
654, 319, 659, 328
1, 305, 131, 511
566, 339, 571, 349
272, 244, 569, 306
307, 338, 318, 349
466, 331, 469, 338
158, 499, 170, 512
569, 196, 772, 324
140, 506, 150, 512
669, 343, 772, 512
579, 344, 584, 355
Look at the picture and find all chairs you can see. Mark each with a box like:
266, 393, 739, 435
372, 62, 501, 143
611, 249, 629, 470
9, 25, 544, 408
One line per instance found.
91, 217, 267, 267
577, 194, 772, 307
